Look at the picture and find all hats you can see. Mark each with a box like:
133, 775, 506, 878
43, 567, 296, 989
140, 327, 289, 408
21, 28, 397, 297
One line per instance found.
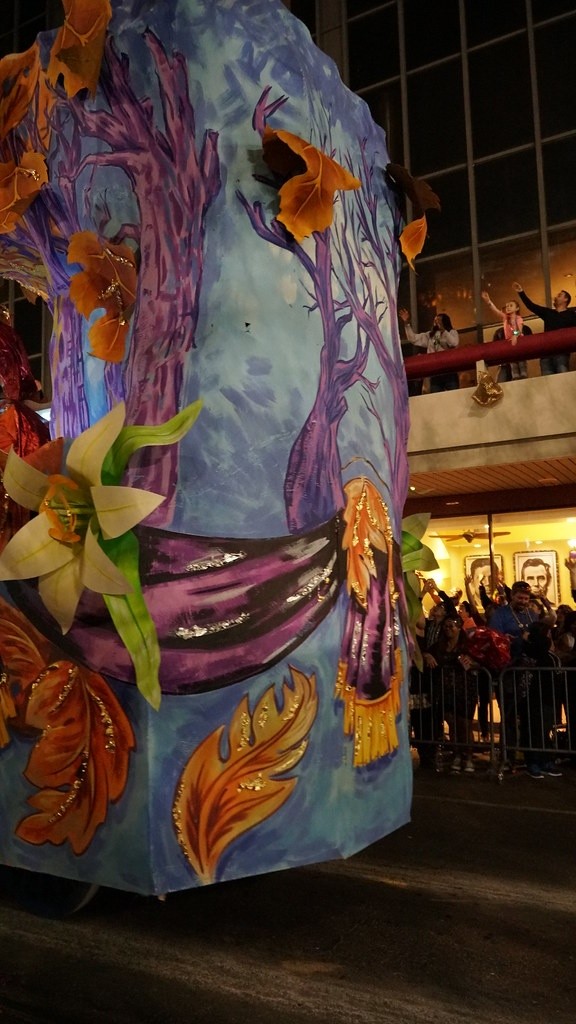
512, 581, 532, 594
462, 601, 472, 617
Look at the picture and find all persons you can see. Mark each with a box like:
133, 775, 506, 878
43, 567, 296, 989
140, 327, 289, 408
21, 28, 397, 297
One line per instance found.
408, 559, 576, 779
481, 291, 528, 380
398, 307, 460, 393
512, 280, 576, 376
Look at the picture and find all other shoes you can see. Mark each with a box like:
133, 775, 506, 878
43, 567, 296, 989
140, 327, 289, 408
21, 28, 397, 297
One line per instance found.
512, 377, 519, 380
520, 375, 527, 379
524, 770, 544, 778
540, 769, 563, 777
451, 758, 462, 770
463, 759, 474, 772
482, 728, 490, 744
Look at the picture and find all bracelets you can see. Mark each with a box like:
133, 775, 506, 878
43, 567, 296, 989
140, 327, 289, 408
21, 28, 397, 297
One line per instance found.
513, 331, 520, 335
